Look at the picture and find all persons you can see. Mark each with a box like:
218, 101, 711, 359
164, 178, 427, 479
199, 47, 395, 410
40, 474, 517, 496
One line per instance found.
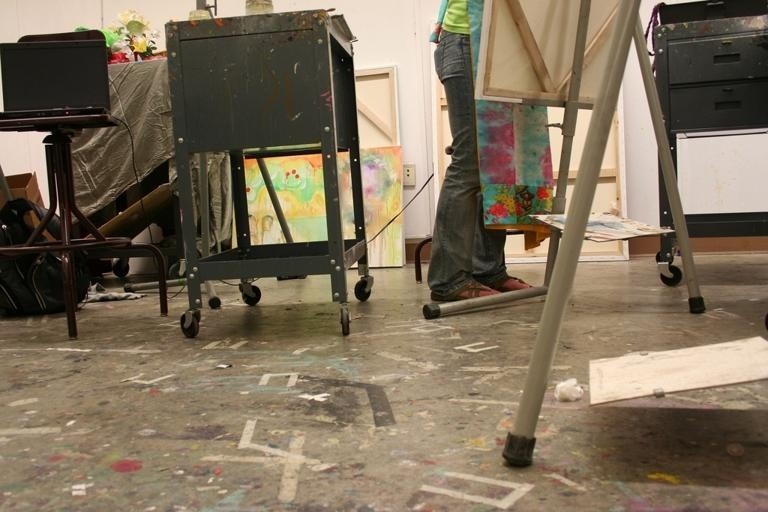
427, 0, 554, 301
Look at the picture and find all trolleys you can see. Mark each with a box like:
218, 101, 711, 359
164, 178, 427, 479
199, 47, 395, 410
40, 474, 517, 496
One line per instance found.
652, 0, 768, 334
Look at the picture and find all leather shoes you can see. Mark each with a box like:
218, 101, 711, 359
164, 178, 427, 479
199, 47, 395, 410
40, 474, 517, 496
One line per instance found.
431, 276, 534, 301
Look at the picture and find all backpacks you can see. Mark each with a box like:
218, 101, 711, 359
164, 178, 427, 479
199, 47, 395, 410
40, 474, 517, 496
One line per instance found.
1, 199, 89, 314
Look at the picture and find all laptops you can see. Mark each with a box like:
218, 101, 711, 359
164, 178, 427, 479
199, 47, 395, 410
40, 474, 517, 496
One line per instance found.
0, 39, 111, 119
